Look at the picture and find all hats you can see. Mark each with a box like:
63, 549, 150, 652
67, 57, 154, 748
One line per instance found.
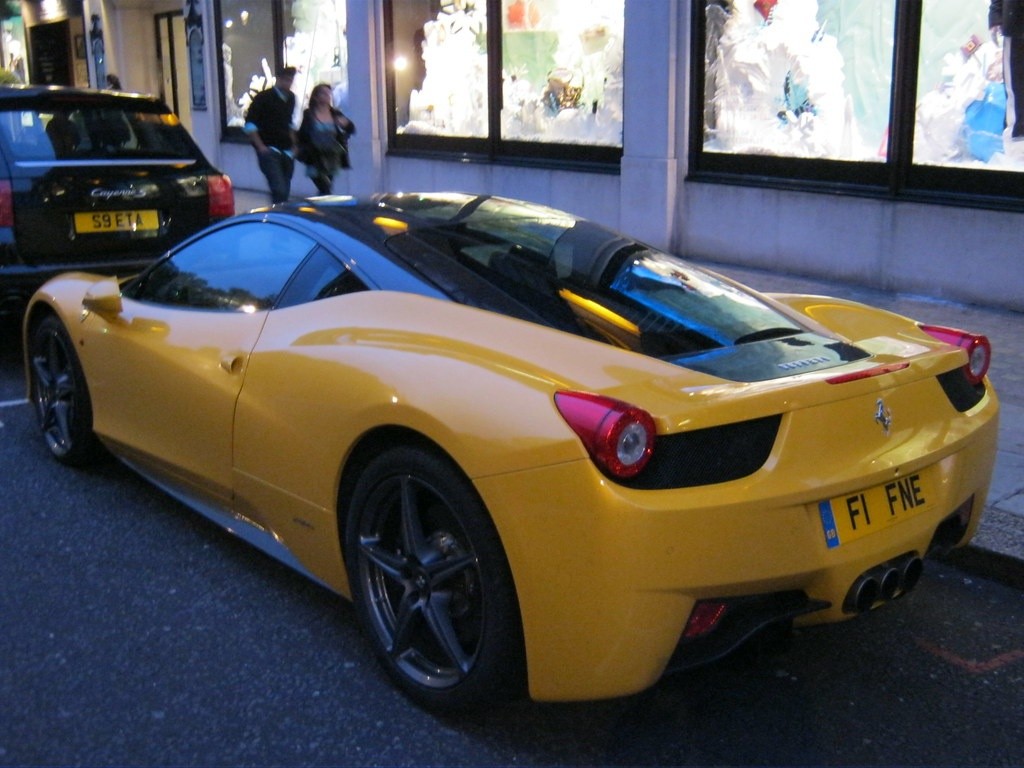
277, 67, 296, 77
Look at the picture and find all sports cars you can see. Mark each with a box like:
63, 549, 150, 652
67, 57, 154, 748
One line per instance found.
22, 190, 1001, 722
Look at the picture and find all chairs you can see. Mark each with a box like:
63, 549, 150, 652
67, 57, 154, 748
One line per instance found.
504, 239, 557, 283
96, 117, 132, 148
45, 120, 81, 150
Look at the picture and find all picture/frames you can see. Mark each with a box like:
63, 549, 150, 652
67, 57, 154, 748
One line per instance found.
74, 35, 86, 59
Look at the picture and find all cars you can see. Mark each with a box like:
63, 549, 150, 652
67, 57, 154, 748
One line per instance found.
1, 82, 238, 343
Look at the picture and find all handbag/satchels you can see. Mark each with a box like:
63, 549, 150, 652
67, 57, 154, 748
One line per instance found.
332, 108, 349, 169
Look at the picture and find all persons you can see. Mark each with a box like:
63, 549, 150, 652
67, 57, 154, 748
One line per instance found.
243, 67, 297, 204
106, 75, 122, 91
296, 84, 357, 195
